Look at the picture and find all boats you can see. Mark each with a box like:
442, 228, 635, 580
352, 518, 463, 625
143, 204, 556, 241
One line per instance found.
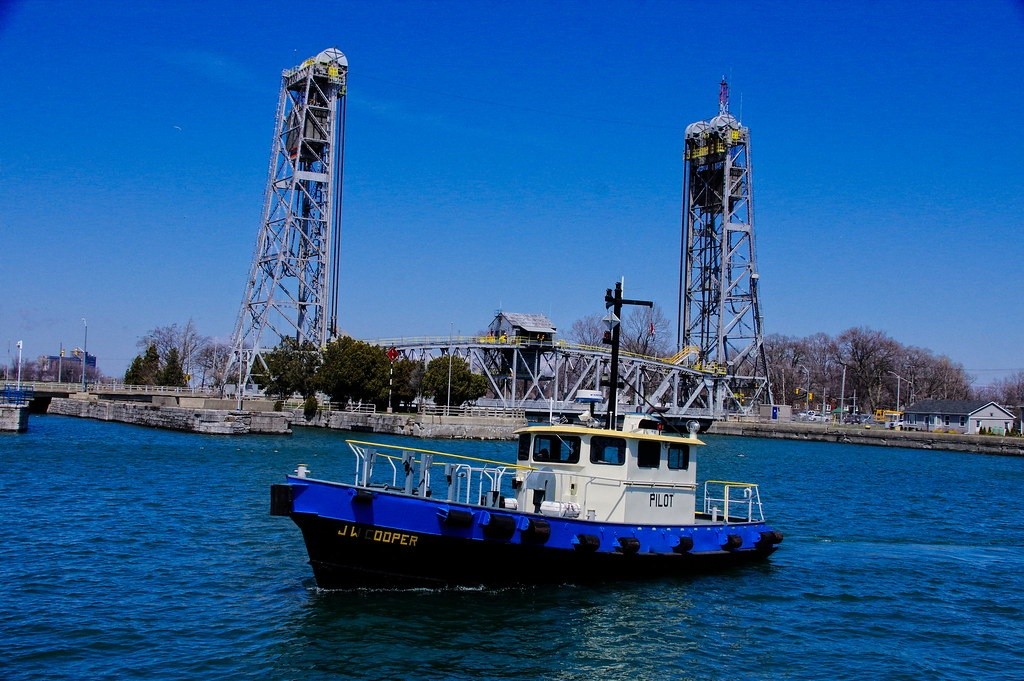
269, 281, 784, 585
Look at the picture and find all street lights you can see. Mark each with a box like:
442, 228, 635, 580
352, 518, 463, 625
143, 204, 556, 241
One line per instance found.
447, 322, 453, 417
81, 318, 87, 391
799, 365, 809, 421
888, 371, 900, 421
